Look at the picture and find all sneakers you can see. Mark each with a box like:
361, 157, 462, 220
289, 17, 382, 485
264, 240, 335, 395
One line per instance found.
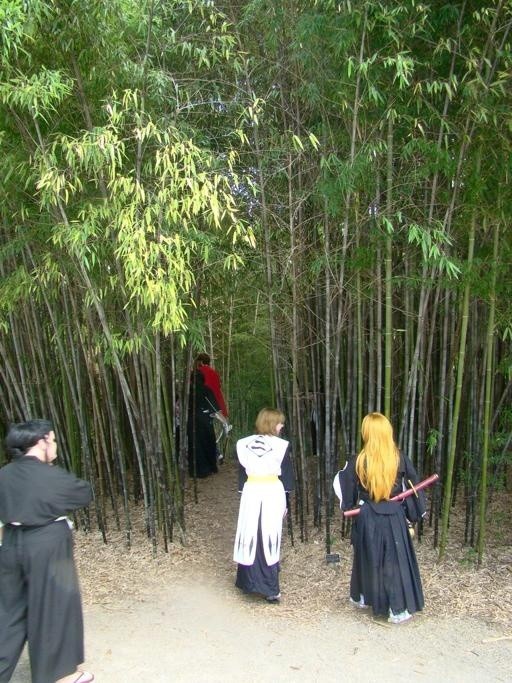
352, 594, 370, 610
55, 669, 94, 683
387, 609, 411, 624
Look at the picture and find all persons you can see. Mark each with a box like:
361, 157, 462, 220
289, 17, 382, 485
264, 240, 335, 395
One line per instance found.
235, 407, 294, 606
175, 354, 229, 481
0, 420, 95, 683
332, 412, 427, 623
